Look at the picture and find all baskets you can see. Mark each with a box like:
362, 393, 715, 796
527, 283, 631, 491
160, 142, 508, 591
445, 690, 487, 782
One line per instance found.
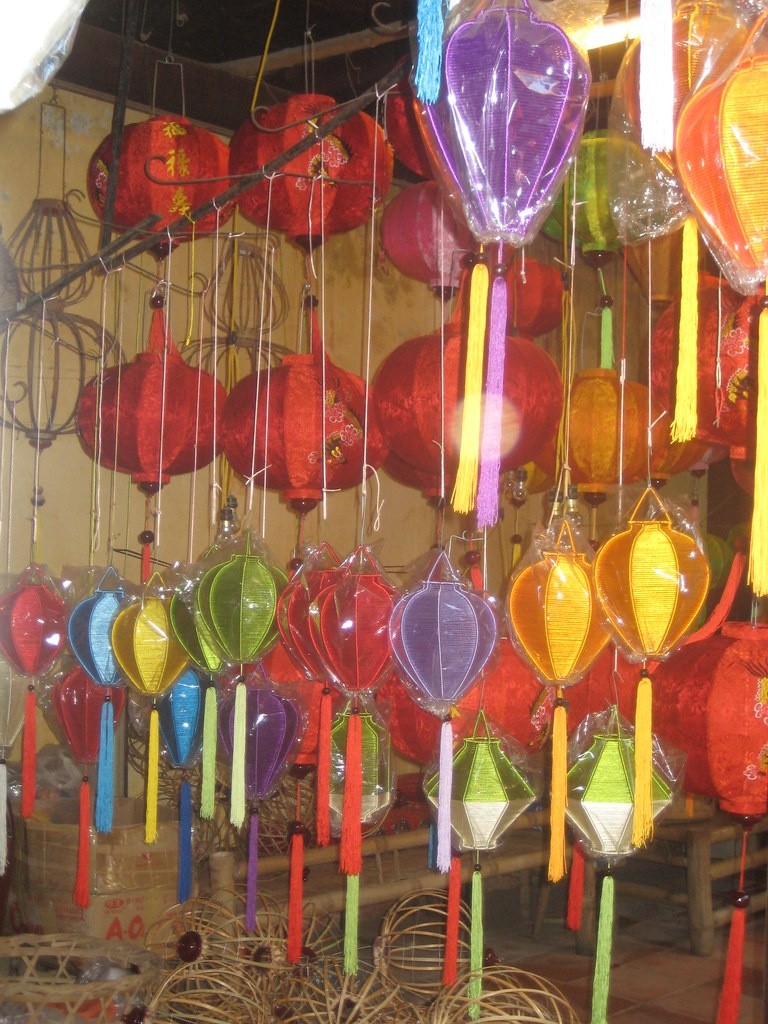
0, 932, 161, 1024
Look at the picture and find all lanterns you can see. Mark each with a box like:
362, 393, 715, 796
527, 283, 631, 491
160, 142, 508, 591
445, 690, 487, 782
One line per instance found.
0, 0, 768, 1024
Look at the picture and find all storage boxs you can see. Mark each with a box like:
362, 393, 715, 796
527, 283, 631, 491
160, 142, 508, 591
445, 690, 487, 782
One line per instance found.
5, 797, 197, 973
